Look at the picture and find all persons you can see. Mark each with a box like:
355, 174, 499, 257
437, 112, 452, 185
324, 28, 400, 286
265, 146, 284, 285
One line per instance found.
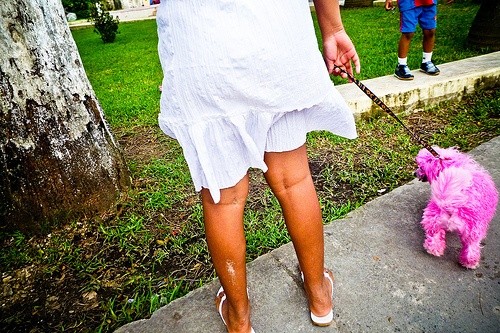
148, 0, 361, 333
384, 0, 440, 80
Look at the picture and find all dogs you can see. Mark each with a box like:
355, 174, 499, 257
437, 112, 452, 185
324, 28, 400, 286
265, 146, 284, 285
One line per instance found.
414, 145, 499, 269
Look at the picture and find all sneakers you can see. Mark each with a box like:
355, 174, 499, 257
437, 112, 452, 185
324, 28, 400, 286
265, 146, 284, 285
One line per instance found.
394, 64, 414, 80
420, 61, 440, 75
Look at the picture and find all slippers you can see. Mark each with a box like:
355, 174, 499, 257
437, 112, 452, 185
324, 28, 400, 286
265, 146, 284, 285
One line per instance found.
301, 265, 338, 327
217, 286, 255, 332
